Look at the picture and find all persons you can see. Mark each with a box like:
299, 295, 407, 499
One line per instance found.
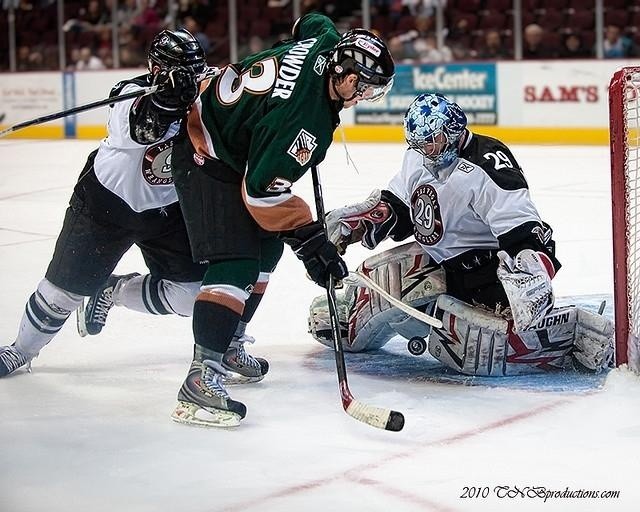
1, 27, 222, 378
0, 1, 640, 71
307, 91, 616, 378
171, 12, 397, 422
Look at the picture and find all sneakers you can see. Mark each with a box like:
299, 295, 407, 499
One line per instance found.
1, 342, 31, 381
86, 269, 119, 335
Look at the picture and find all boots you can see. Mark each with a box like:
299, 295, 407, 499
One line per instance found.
222, 321, 270, 376
178, 346, 247, 415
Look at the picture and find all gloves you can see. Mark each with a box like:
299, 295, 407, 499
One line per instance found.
304, 241, 350, 293
148, 63, 201, 114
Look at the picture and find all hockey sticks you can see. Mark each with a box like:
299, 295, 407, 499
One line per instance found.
311, 165, 406, 432
0, 67, 220, 138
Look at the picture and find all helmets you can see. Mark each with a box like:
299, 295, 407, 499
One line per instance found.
325, 24, 394, 101
406, 89, 468, 159
147, 27, 206, 76
